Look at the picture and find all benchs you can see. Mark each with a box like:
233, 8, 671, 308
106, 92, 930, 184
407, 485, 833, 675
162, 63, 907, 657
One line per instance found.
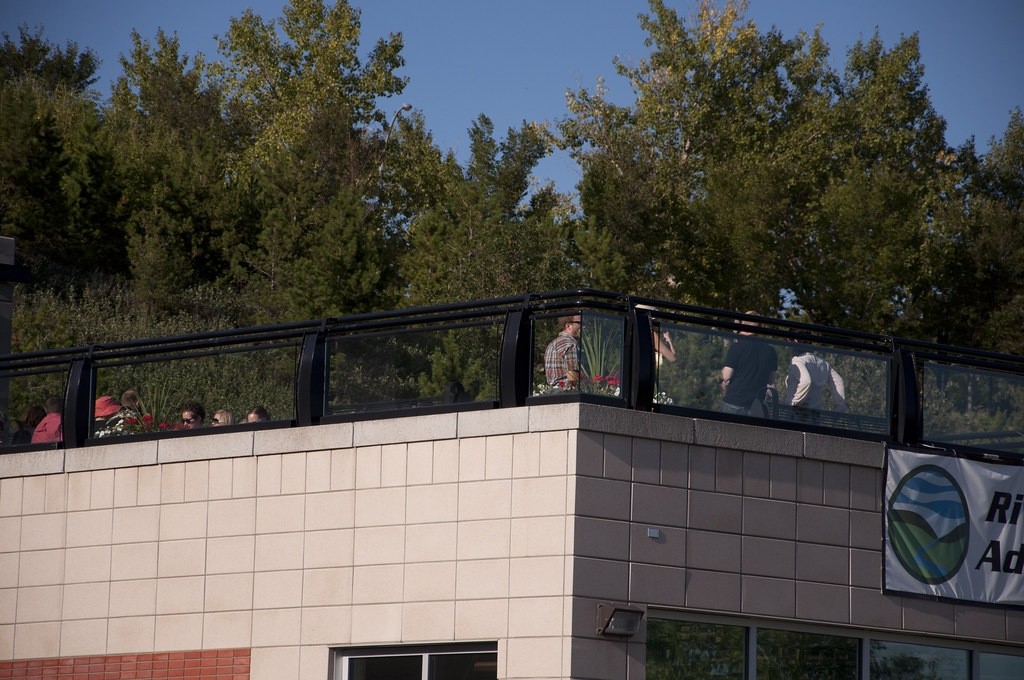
327, 379, 470, 417
760, 387, 888, 434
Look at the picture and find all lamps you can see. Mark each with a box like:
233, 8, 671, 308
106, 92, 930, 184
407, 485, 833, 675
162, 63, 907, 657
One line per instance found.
595, 600, 644, 642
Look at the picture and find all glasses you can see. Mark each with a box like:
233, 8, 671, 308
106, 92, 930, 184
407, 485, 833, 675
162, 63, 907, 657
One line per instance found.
182, 417, 194, 424
212, 419, 224, 422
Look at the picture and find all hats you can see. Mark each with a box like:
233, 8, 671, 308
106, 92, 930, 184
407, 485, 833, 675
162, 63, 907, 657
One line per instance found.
94, 396, 123, 418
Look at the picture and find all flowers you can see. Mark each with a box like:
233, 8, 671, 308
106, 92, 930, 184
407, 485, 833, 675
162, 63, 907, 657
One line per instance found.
88, 386, 194, 439
531, 366, 674, 407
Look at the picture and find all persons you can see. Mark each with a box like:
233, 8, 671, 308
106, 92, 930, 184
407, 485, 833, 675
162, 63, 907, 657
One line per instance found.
614, 305, 845, 419
544, 310, 587, 389
12, 389, 268, 445
